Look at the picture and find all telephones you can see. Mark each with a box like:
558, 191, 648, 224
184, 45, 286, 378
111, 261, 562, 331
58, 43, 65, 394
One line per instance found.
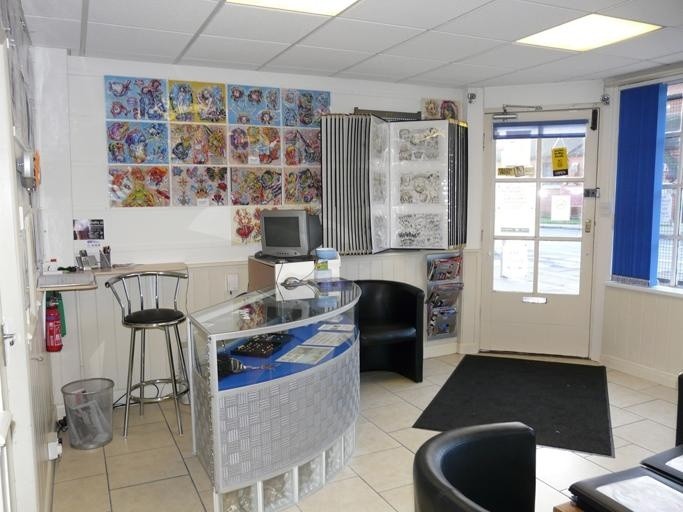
75, 249, 100, 271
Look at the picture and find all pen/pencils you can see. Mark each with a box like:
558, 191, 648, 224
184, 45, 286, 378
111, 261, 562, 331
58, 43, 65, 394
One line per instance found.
100, 246, 111, 268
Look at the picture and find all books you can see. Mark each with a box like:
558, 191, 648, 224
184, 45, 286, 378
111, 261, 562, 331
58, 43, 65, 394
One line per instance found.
425, 249, 465, 336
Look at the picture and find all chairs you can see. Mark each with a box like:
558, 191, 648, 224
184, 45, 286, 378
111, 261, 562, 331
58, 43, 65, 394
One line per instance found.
410, 417, 541, 512
351, 279, 425, 386
106, 267, 194, 439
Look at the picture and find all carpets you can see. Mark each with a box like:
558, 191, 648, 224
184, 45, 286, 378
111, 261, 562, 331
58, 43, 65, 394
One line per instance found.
413, 344, 614, 459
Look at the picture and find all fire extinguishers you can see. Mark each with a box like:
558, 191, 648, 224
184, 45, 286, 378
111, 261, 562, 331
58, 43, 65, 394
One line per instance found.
46, 295, 63, 350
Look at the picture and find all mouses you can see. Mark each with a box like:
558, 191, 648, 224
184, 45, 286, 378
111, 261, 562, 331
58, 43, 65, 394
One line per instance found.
285, 277, 301, 285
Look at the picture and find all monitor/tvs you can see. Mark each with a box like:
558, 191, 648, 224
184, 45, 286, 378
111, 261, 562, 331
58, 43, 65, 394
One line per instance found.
260, 210, 323, 261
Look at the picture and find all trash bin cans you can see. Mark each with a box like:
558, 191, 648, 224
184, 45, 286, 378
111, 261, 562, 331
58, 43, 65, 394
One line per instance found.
61, 379, 114, 450
